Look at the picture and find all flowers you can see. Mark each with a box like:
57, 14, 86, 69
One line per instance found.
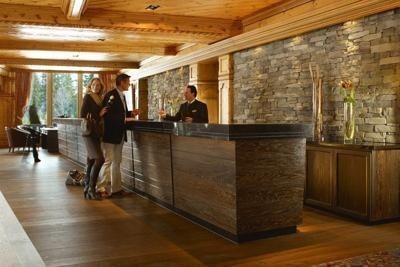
340, 80, 355, 138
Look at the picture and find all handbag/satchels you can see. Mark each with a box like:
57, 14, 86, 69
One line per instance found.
81, 118, 102, 139
65, 170, 85, 186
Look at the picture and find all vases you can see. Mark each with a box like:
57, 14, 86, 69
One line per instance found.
343, 102, 355, 143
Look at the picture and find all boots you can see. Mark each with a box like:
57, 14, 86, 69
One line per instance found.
83, 163, 102, 200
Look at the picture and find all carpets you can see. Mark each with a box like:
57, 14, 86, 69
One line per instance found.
313, 247, 399, 267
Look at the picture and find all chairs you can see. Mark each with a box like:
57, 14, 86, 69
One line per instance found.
4, 124, 41, 153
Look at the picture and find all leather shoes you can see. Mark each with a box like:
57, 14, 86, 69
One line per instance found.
98, 190, 125, 199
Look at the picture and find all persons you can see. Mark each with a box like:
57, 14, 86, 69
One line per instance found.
82, 77, 110, 199
94, 74, 144, 194
157, 85, 207, 123
